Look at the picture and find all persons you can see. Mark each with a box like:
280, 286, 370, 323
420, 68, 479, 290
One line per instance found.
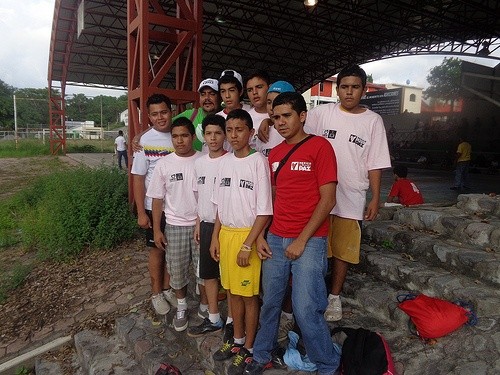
114, 130, 128, 170
207, 107, 274, 375
449, 134, 472, 190
416, 154, 429, 169
486, 158, 499, 175
243, 93, 344, 375
129, 63, 392, 375
386, 164, 424, 208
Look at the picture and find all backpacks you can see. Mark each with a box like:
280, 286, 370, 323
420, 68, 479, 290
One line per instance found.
331, 324, 394, 375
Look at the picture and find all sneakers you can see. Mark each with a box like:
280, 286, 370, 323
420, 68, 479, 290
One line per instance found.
212, 338, 245, 361
227, 348, 253, 375
188, 318, 225, 337
198, 307, 209, 319
152, 293, 170, 315
243, 360, 267, 375
324, 294, 342, 321
223, 321, 234, 343
163, 289, 178, 308
272, 357, 284, 369
277, 311, 294, 341
173, 308, 189, 331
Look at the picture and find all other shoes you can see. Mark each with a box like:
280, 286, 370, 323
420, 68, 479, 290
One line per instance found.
196, 283, 201, 295
450, 187, 461, 190
217, 293, 226, 300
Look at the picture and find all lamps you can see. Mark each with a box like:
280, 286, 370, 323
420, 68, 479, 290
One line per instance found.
215, 5, 226, 23
478, 41, 490, 57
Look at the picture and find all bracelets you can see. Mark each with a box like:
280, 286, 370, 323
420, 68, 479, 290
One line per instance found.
240, 242, 252, 252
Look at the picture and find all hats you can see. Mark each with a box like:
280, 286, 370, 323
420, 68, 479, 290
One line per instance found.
219, 70, 243, 88
267, 81, 295, 93
197, 78, 219, 93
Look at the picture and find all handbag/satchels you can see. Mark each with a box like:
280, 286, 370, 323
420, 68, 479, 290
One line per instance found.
397, 293, 471, 345
